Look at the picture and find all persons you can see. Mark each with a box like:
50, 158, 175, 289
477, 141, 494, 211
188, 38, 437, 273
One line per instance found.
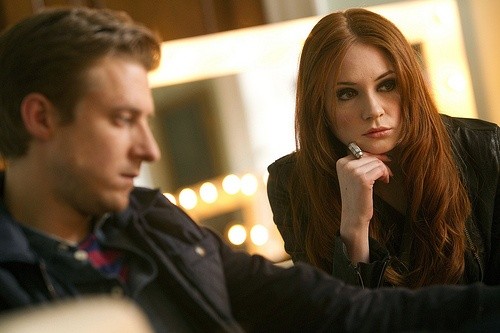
267, 8, 500, 290
0, 7, 500, 333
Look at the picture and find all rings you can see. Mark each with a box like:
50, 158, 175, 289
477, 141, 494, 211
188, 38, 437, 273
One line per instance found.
347, 142, 364, 160
